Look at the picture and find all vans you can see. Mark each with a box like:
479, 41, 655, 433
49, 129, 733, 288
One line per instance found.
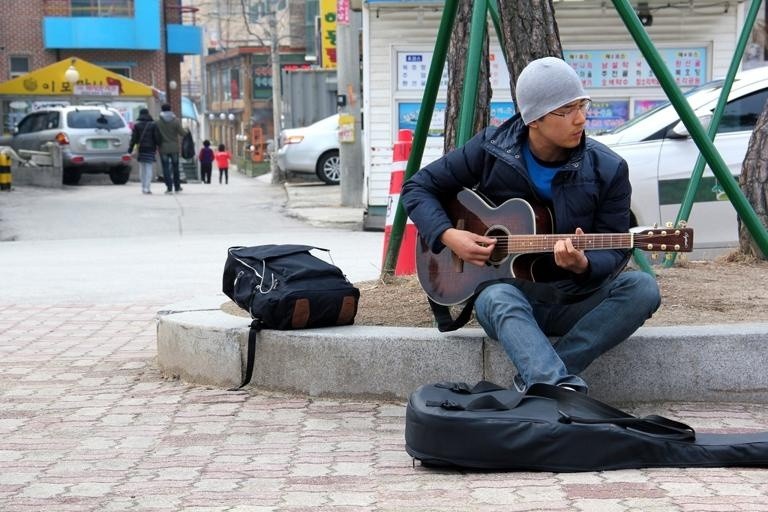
0, 105, 135, 185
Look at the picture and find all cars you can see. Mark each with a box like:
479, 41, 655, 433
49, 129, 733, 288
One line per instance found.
275, 107, 364, 186
574, 66, 767, 262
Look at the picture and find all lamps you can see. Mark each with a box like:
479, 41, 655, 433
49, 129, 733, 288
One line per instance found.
65, 59, 81, 82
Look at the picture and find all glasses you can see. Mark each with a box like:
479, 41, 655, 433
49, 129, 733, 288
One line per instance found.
550, 98, 592, 120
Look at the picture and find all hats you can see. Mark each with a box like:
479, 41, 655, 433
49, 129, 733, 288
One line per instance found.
515, 57, 590, 125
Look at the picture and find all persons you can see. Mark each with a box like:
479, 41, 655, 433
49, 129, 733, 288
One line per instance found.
155, 102, 189, 193
127, 110, 162, 194
199, 139, 214, 184
216, 144, 231, 184
403, 57, 663, 399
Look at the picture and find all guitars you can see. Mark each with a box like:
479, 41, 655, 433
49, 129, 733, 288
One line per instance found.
416, 185, 693, 306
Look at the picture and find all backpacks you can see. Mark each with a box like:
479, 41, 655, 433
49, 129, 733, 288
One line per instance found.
222, 245, 360, 330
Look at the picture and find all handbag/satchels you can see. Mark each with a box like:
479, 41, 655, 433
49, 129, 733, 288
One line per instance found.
181, 135, 193, 159
132, 147, 139, 159
